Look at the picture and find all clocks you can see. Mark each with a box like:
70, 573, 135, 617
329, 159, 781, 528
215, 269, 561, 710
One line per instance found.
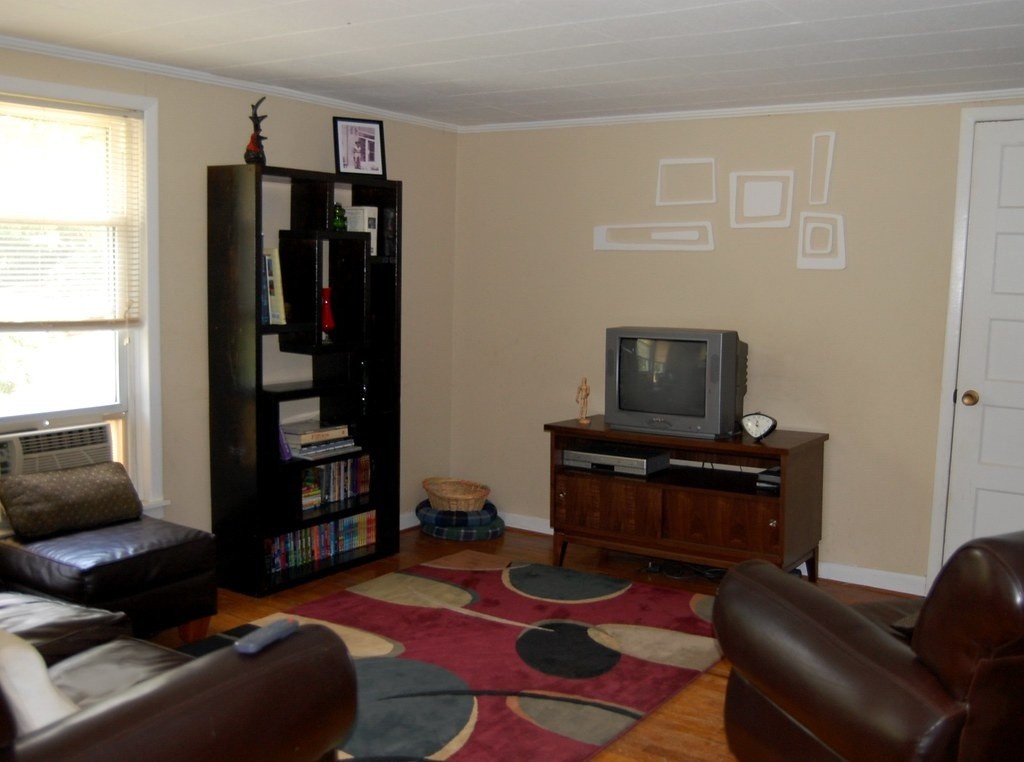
742, 413, 777, 441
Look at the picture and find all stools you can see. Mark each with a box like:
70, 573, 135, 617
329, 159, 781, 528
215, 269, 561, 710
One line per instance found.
0, 514, 217, 636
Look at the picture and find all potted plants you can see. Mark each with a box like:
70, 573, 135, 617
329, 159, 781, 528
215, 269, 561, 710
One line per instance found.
244, 97, 268, 165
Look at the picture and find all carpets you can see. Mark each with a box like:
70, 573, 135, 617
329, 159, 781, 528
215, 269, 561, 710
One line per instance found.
178, 549, 725, 762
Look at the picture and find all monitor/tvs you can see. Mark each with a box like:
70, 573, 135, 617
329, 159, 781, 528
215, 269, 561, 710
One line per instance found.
605, 326, 748, 441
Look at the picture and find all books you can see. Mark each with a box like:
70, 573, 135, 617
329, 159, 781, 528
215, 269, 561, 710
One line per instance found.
278, 419, 362, 460
339, 510, 377, 552
302, 456, 371, 512
272, 522, 335, 573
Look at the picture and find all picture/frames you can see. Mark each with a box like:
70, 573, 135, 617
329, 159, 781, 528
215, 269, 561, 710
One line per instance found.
332, 116, 387, 179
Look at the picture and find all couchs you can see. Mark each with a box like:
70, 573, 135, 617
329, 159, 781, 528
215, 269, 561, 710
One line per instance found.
0, 594, 357, 762
710, 530, 1024, 762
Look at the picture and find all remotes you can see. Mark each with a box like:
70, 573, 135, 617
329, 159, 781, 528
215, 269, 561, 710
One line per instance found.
234, 617, 298, 655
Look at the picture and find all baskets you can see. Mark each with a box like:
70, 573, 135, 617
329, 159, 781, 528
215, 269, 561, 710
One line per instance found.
422, 477, 490, 512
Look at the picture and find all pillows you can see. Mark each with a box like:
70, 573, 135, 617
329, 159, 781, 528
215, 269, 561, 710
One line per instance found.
0, 461, 142, 545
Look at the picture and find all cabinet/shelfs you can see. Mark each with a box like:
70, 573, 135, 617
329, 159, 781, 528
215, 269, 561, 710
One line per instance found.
542, 414, 830, 583
207, 164, 402, 597
